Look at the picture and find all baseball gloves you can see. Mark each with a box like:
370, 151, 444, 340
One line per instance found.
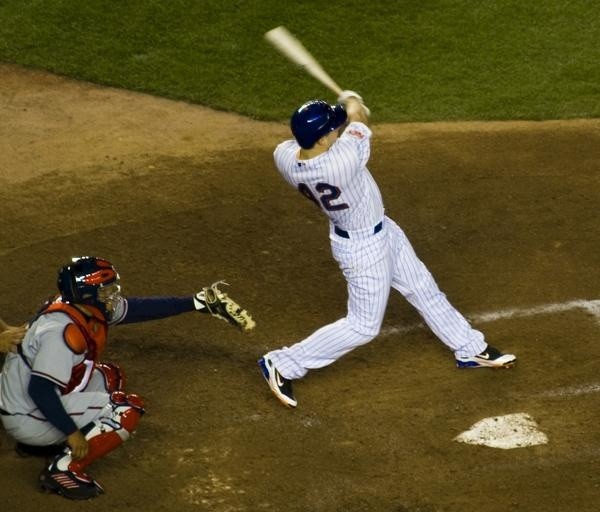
193, 278, 257, 332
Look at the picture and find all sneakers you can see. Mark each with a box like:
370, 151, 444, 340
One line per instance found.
456, 346, 517, 369
256, 354, 298, 408
15, 441, 65, 458
31, 471, 106, 501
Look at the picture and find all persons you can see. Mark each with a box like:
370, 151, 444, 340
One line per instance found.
257, 90, 517, 408
0, 256, 255, 499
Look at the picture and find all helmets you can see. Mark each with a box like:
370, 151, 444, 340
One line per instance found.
57, 256, 121, 321
291, 100, 348, 148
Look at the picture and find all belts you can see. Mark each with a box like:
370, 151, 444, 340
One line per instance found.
335, 221, 382, 238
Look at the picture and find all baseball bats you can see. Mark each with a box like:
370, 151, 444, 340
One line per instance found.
265, 25, 371, 116
